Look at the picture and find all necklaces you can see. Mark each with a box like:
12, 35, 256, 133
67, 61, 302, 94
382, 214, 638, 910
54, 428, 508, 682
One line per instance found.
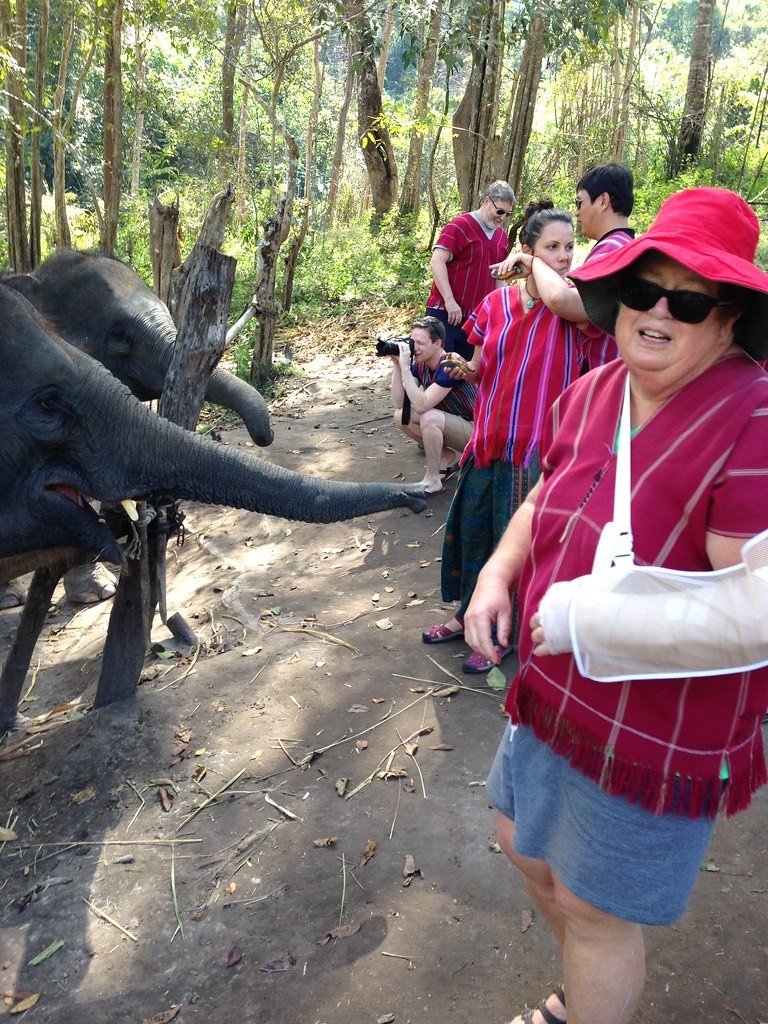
525, 280, 541, 309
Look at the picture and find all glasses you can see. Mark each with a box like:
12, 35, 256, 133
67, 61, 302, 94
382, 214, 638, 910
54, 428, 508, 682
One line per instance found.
607, 273, 737, 324
413, 318, 441, 339
487, 195, 513, 217
575, 191, 613, 210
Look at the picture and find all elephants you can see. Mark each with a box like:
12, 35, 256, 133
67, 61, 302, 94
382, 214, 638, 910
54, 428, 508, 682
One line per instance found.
0, 249, 275, 609
0, 283, 426, 576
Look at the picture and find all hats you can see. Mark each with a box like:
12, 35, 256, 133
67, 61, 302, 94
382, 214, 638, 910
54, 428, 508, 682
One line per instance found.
568, 188, 768, 361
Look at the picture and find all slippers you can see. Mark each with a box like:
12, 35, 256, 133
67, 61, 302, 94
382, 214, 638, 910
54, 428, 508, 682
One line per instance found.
438, 459, 460, 483
422, 487, 446, 498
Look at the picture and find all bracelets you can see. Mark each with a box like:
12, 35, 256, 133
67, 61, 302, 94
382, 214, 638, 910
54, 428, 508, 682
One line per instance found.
530, 255, 538, 273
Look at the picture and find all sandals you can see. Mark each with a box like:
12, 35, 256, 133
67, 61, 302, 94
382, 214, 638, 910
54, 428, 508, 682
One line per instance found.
511, 984, 567, 1024
421, 615, 465, 643
462, 641, 513, 672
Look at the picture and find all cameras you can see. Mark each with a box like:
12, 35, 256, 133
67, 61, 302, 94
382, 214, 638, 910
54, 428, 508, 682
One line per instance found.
376, 338, 414, 358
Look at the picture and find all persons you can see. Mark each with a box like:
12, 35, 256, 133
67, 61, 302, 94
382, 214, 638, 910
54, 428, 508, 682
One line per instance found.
464, 188, 768, 1024
575, 162, 636, 378
424, 180, 516, 361
389, 316, 478, 496
421, 197, 605, 673
